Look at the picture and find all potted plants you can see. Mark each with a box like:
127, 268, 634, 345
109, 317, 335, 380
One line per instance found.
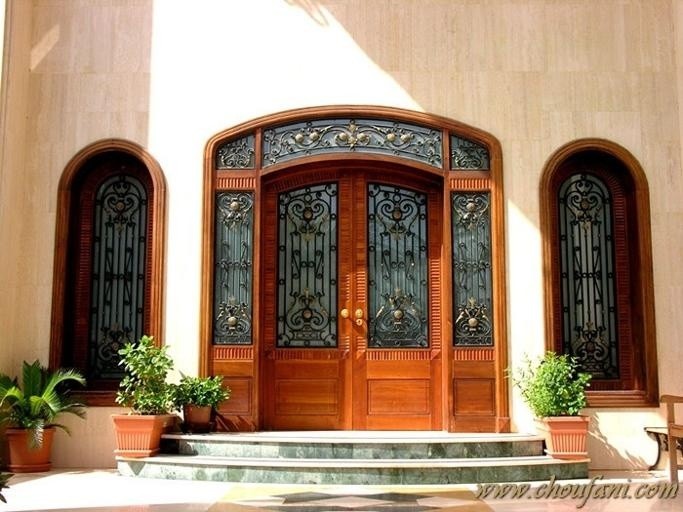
166, 371, 232, 424
110, 334, 176, 458
499, 348, 592, 461
0, 359, 86, 473
